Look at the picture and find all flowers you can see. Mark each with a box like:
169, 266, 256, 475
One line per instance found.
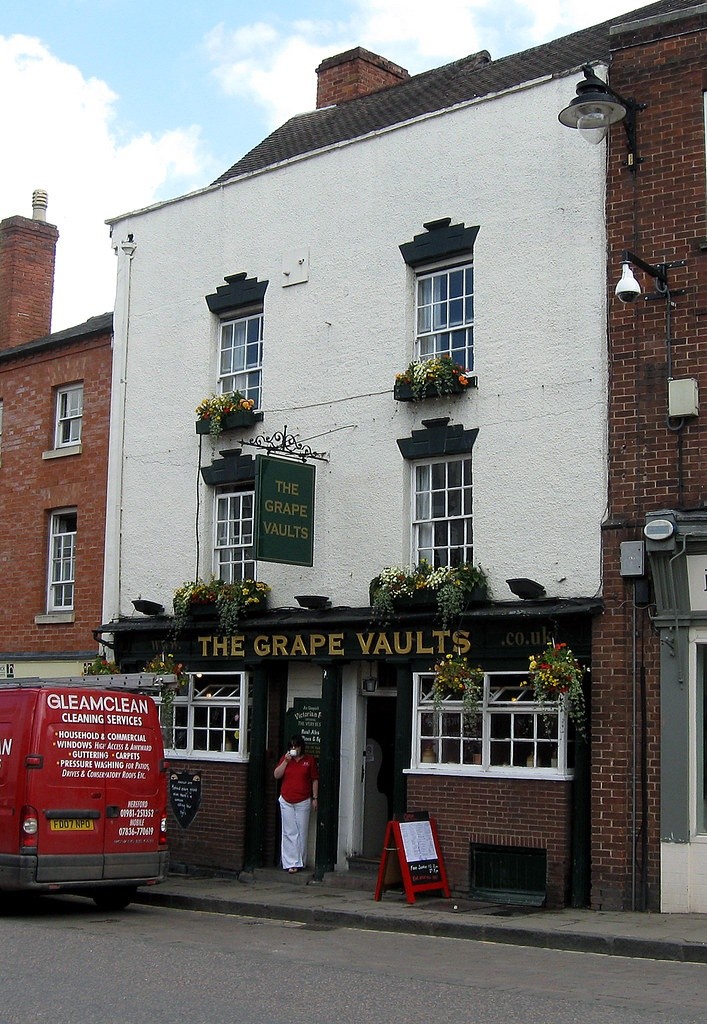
195, 389, 255, 440
141, 650, 188, 727
512, 642, 591, 742
161, 573, 271, 648
81, 654, 121, 676
366, 558, 490, 633
395, 353, 467, 403
428, 654, 485, 742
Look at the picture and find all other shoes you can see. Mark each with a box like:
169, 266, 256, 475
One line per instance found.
289, 867, 297, 874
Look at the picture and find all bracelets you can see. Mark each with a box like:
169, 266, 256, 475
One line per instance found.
312, 796, 318, 800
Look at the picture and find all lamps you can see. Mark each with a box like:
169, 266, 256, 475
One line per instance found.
558, 63, 648, 171
360, 660, 381, 694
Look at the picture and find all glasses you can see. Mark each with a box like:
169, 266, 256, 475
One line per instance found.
290, 744, 300, 747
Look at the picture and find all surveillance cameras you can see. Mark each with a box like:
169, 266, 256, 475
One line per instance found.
615, 263, 641, 303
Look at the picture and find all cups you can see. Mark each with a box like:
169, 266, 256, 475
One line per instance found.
290, 750, 296, 756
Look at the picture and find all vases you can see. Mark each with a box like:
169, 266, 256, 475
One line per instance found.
173, 595, 268, 617
370, 580, 488, 612
196, 408, 255, 434
394, 377, 468, 401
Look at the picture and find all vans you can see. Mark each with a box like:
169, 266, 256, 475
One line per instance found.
0, 671, 181, 910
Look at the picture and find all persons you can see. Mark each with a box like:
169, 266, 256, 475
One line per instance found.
273, 735, 319, 874
